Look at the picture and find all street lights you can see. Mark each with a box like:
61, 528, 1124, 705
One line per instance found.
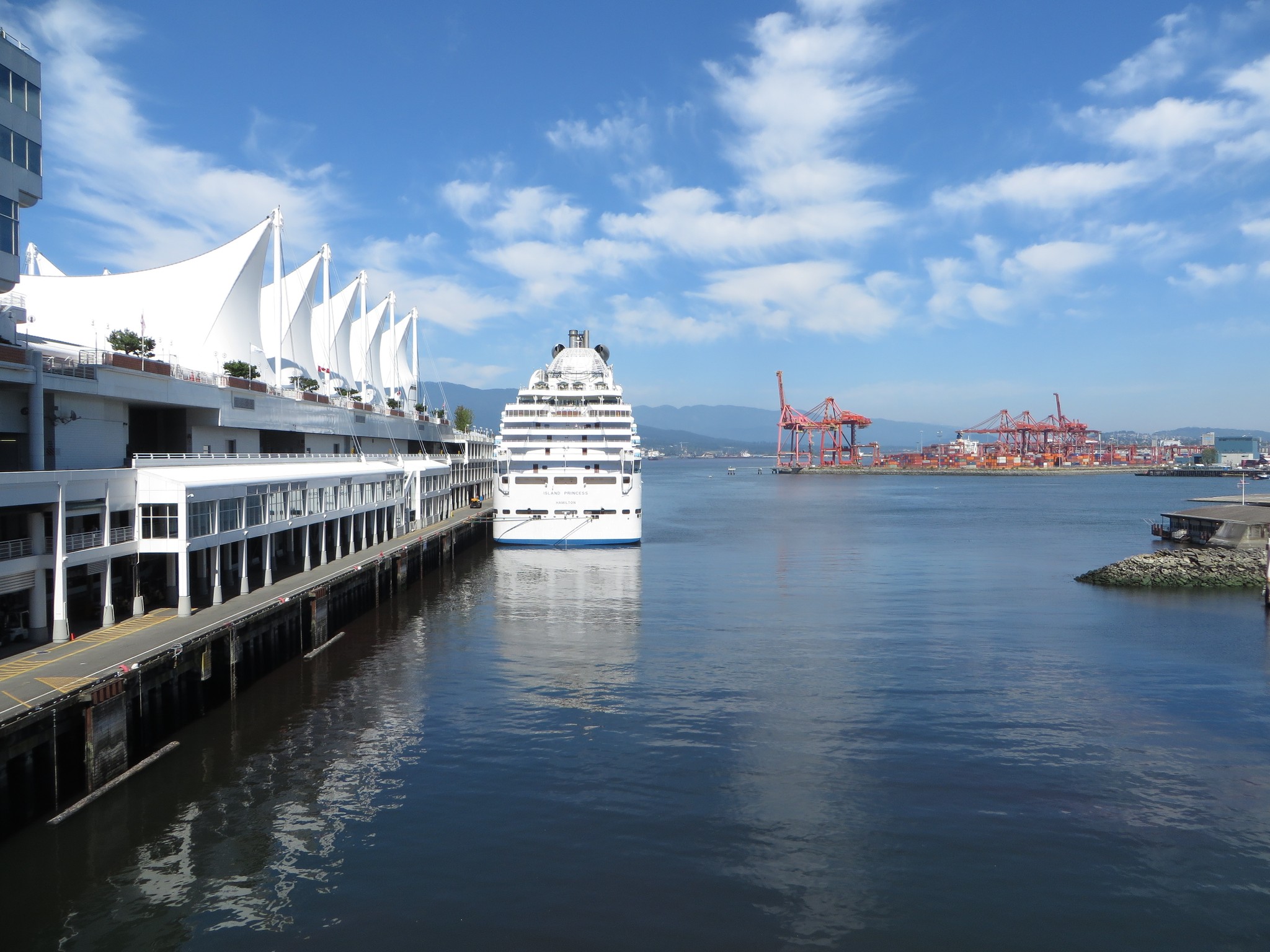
258, 362, 268, 383
986, 432, 990, 453
214, 351, 227, 375
1019, 430, 1023, 456
465, 424, 493, 439
91, 320, 109, 363
916, 442, 919, 453
1057, 438, 1061, 468
158, 337, 173, 365
293, 372, 301, 391
1155, 430, 1159, 462
1099, 432, 1103, 461
937, 431, 943, 468
968, 429, 972, 441
435, 408, 438, 418
426, 405, 428, 416
1075, 432, 1078, 451
1110, 438, 1114, 465
445, 410, 448, 419
1025, 431, 1028, 454
399, 398, 404, 411
413, 402, 416, 414
321, 378, 329, 396
920, 430, 923, 454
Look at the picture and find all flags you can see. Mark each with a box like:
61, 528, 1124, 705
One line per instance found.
251, 344, 265, 354
141, 316, 145, 334
442, 404, 444, 408
318, 366, 330, 373
423, 396, 425, 406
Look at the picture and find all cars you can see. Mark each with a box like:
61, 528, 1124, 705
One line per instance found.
470, 501, 482, 508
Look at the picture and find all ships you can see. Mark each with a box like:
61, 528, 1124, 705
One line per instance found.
492, 328, 645, 545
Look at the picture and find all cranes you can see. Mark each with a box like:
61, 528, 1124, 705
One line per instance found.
956, 393, 1100, 455
776, 371, 872, 468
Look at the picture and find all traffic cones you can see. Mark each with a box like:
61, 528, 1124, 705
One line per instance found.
69, 633, 75, 641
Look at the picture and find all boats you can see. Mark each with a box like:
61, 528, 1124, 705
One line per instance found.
647, 448, 663, 460
1258, 474, 1270, 480
